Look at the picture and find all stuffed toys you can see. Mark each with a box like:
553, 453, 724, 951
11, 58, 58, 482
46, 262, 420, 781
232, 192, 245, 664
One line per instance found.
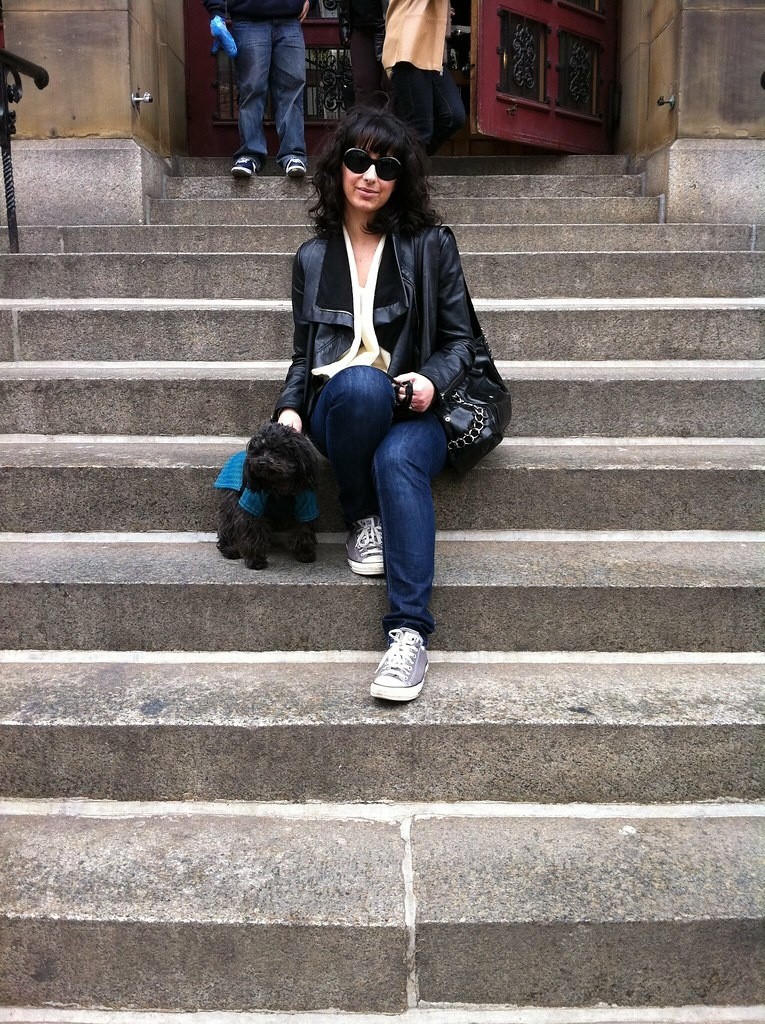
209, 15, 238, 57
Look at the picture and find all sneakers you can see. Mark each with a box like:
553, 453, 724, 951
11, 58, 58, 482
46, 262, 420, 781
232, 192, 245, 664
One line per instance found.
285, 157, 307, 178
345, 515, 385, 575
230, 155, 265, 178
370, 627, 429, 701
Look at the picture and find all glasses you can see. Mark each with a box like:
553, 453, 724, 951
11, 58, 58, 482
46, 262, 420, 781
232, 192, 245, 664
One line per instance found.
341, 147, 402, 181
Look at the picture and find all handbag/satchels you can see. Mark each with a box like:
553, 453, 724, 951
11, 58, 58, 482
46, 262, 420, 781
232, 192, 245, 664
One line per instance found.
374, 19, 386, 62
413, 225, 512, 481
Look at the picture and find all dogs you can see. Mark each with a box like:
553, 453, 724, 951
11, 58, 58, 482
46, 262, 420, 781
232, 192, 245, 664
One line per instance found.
216, 422, 332, 570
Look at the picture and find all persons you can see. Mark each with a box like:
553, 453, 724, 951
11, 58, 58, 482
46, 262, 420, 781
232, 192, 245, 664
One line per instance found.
381, 0, 465, 157
350, 0, 384, 104
203, 0, 312, 178
270, 105, 513, 702
